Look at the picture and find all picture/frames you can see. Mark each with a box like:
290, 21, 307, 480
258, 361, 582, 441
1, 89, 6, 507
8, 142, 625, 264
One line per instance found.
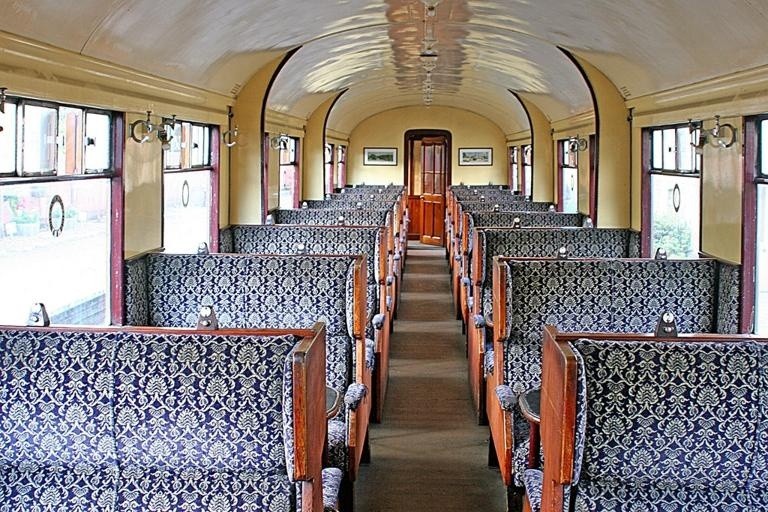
362, 147, 398, 166
458, 146, 494, 166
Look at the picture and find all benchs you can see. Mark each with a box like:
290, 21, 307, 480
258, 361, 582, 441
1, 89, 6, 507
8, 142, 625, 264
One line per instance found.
2, 183, 410, 511
443, 183, 765, 511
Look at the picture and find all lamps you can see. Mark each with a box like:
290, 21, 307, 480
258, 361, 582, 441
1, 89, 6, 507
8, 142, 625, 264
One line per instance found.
221, 106, 251, 149
129, 102, 179, 150
686, 114, 739, 156
418, 0, 441, 71
270, 132, 289, 155
568, 132, 588, 155
